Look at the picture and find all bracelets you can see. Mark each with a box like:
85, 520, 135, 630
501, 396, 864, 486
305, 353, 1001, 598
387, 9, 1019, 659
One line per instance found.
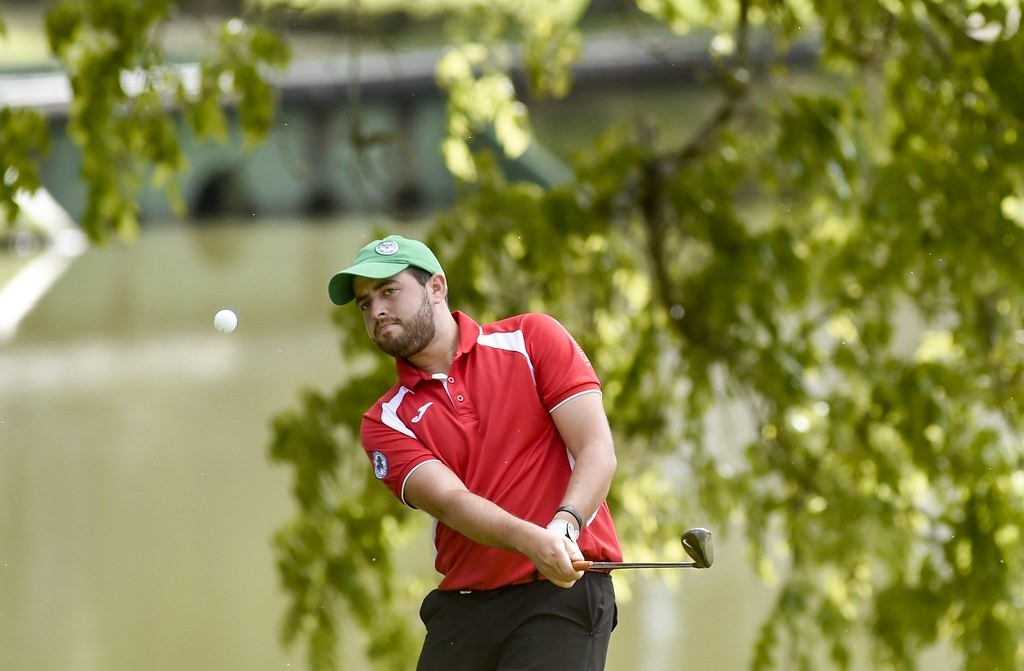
555, 506, 582, 531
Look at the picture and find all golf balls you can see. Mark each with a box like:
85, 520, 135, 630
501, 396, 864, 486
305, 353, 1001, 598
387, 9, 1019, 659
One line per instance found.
214, 310, 237, 334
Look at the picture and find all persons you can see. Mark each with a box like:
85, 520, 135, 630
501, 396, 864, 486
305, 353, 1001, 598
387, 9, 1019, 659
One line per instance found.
328, 233, 624, 671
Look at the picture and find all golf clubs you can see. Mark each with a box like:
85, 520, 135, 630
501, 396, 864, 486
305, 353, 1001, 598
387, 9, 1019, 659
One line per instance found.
571, 528, 714, 571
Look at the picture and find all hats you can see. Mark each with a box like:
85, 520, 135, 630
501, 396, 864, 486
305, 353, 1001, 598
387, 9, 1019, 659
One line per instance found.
328, 234, 447, 306
